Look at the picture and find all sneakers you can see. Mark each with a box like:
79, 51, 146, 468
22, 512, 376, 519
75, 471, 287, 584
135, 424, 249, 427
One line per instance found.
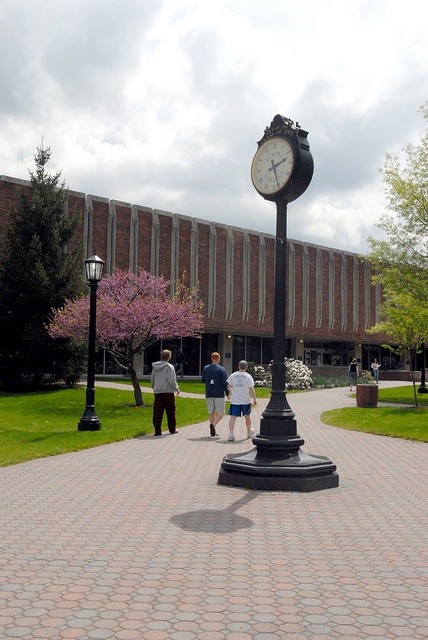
170, 428, 178, 433
210, 434, 221, 439
247, 427, 255, 438
210, 423, 216, 436
154, 431, 164, 436
228, 434, 235, 442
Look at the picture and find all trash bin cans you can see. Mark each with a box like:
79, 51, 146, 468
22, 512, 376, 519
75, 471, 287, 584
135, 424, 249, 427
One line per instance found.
356, 384, 379, 407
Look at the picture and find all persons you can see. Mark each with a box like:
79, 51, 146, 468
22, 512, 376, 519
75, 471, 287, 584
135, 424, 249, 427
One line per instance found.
199, 351, 229, 438
346, 357, 360, 393
370, 357, 383, 385
150, 350, 181, 438
226, 358, 258, 442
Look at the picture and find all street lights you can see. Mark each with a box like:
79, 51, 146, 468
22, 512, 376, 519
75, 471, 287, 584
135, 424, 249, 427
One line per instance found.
77, 253, 106, 431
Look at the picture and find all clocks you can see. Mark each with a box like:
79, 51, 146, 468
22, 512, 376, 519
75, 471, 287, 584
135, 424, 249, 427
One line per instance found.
251, 114, 314, 203
